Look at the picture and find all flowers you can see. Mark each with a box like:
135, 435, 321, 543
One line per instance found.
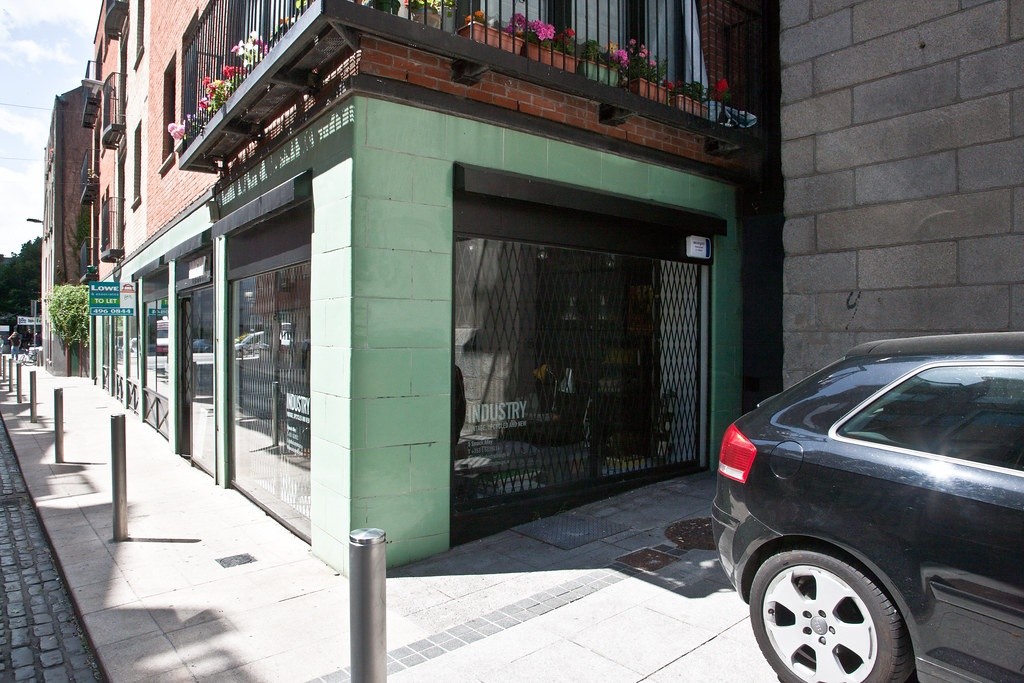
167, 0, 307, 143
404, 0, 732, 103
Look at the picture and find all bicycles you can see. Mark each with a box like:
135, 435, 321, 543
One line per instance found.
19, 348, 37, 366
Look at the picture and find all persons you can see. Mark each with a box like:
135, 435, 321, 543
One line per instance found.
0, 335, 4, 353
7, 332, 21, 360
36, 330, 42, 346
24, 328, 32, 346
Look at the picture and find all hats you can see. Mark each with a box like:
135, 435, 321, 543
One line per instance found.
12, 332, 19, 335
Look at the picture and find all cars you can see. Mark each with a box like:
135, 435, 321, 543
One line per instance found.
194, 353, 213, 397
235, 331, 264, 359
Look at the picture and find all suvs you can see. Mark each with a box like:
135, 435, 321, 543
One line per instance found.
711, 332, 1024, 683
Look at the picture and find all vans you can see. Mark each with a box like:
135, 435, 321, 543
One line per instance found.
125, 338, 169, 370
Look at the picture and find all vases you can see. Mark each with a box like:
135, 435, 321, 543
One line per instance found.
578, 58, 624, 86
524, 41, 581, 73
454, 20, 526, 55
408, 8, 441, 30
669, 94, 708, 118
369, 0, 401, 15
627, 78, 671, 104
